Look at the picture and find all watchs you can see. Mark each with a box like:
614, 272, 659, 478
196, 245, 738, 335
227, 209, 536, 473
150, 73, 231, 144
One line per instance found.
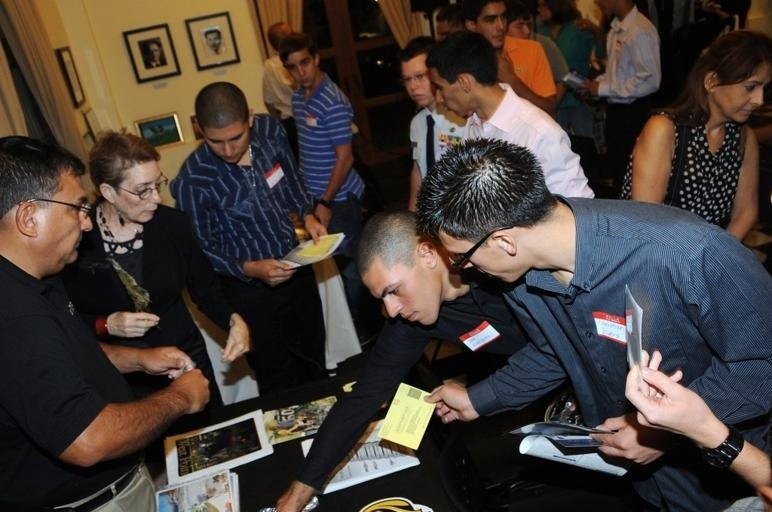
701, 423, 743, 472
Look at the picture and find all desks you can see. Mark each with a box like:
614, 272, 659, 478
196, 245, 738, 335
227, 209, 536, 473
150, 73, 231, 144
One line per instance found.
148, 373, 466, 511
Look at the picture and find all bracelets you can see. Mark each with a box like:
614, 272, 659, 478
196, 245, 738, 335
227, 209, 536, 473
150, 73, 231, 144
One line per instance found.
320, 199, 336, 210
95, 316, 110, 338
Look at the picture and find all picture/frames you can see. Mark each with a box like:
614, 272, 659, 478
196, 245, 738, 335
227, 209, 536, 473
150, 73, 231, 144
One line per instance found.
183, 11, 240, 73
120, 22, 181, 86
52, 45, 87, 109
133, 110, 185, 151
81, 106, 104, 152
188, 112, 205, 144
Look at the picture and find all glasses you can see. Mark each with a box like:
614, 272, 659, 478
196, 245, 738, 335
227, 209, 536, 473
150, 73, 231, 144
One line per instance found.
19, 198, 91, 218
448, 224, 512, 268
119, 174, 169, 199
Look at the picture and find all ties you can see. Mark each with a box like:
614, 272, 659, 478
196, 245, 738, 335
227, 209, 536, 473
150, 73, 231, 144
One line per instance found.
426, 115, 435, 174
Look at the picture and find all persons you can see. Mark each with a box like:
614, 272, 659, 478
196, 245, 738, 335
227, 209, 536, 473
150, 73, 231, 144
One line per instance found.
417, 137, 770, 511
264, 23, 300, 168
398, 0, 772, 212
277, 31, 379, 345
623, 350, 771, 510
270, 209, 589, 511
202, 28, 223, 55
140, 38, 165, 69
618, 29, 771, 240
1, 135, 212, 512
169, 83, 330, 396
58, 129, 251, 483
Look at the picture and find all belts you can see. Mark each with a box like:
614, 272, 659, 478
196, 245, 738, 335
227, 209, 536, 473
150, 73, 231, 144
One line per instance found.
54, 465, 138, 512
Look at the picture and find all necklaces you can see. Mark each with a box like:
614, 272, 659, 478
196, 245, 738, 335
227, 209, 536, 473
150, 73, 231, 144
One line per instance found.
97, 207, 139, 249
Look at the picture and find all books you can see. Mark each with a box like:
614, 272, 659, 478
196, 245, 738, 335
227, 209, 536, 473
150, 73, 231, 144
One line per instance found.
155, 469, 241, 512
261, 396, 341, 446
165, 407, 275, 484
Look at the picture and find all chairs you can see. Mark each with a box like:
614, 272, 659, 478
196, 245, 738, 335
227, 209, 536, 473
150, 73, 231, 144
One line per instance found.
342, 314, 651, 512
